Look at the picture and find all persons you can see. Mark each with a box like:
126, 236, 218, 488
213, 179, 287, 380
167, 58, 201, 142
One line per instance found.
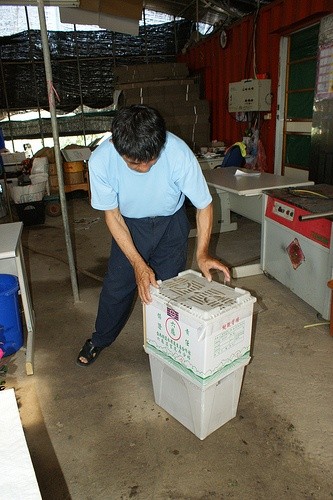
77, 104, 231, 366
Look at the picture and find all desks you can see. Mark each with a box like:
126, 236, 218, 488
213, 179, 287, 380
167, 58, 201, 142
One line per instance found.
197, 157, 224, 170
188, 166, 315, 279
0, 221, 36, 375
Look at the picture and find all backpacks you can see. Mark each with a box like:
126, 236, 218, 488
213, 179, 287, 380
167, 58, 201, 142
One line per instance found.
221, 142, 246, 168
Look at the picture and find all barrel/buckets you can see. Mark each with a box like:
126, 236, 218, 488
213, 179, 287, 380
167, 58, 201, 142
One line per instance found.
0, 274, 24, 357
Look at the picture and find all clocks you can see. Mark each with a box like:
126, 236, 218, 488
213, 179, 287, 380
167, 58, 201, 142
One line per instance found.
219, 30, 228, 49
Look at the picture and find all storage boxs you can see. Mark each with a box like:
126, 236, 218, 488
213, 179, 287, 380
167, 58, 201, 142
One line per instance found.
1, 152, 25, 173
143, 270, 258, 441
18, 202, 44, 224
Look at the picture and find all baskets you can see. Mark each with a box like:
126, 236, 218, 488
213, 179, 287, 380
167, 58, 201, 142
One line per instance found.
1, 152, 85, 203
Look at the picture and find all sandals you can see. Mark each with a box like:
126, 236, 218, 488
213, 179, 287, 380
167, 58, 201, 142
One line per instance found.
77, 338, 98, 367
